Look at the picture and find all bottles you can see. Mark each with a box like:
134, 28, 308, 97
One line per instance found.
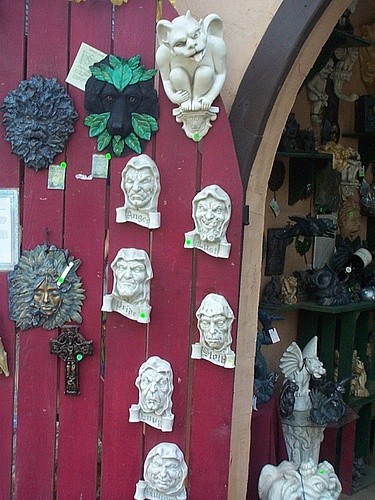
343, 244, 375, 275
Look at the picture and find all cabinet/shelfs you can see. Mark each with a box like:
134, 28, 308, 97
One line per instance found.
274, 27, 374, 208
293, 298, 375, 496
